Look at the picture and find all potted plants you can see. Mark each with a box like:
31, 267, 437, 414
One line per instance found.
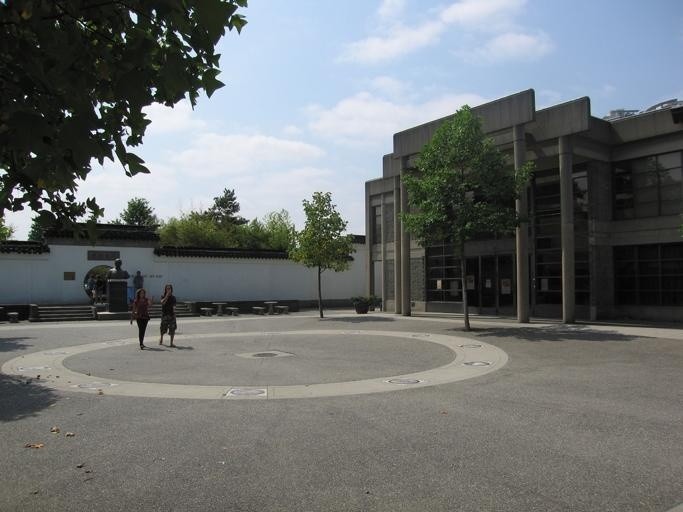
350, 296, 369, 314
368, 295, 380, 311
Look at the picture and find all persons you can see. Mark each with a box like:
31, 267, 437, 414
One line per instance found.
88, 271, 178, 349
108, 259, 130, 279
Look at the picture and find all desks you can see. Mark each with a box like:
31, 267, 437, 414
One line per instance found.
264, 302, 278, 315
212, 303, 227, 316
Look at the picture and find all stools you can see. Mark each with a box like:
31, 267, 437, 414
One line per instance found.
274, 306, 289, 314
200, 307, 215, 316
6, 311, 19, 323
225, 307, 240, 316
252, 307, 266, 315
